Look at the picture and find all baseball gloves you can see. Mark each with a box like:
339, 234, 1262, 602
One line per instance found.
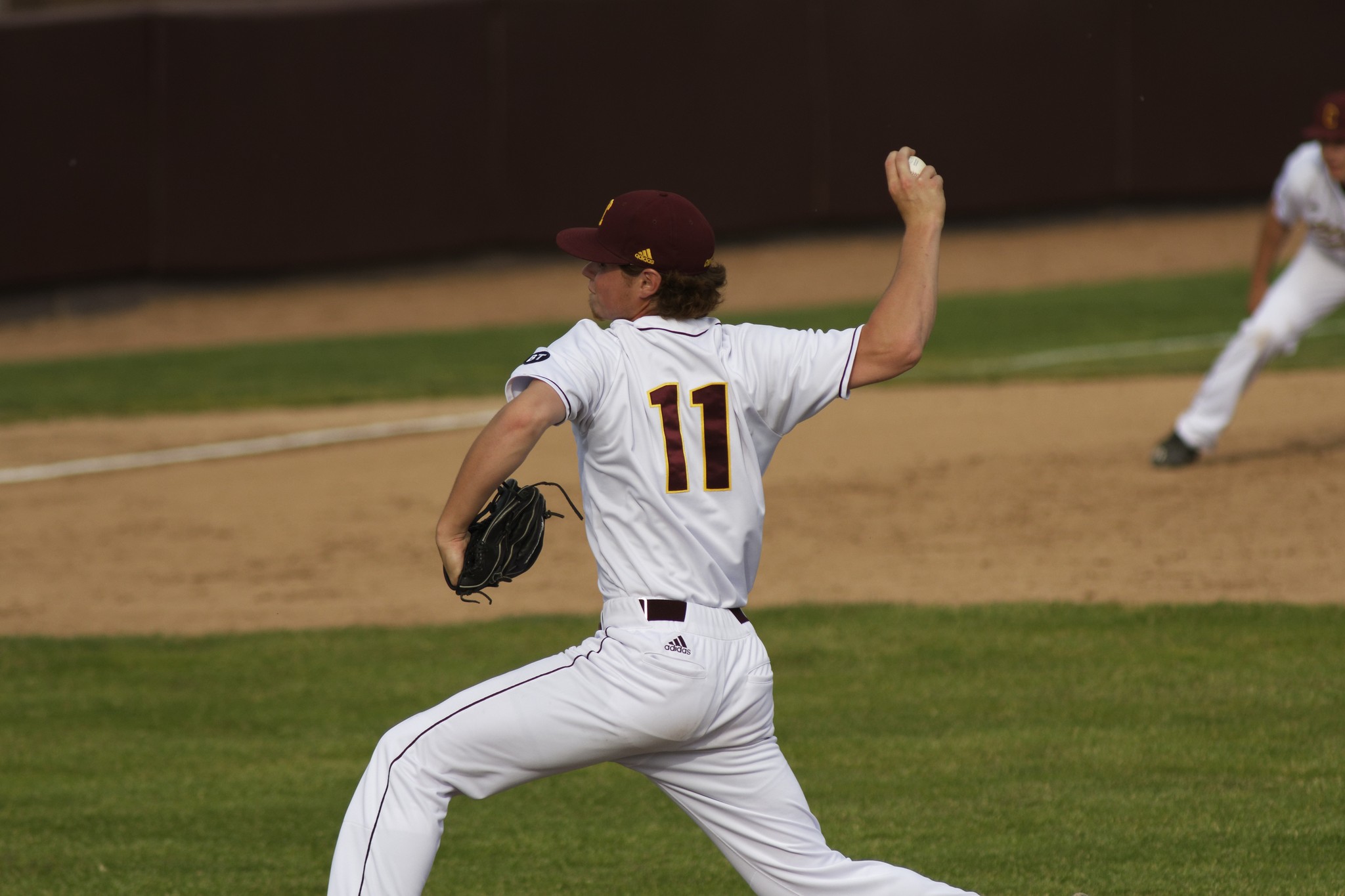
441, 478, 548, 596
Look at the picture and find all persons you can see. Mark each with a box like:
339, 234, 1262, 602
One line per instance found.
326, 146, 983, 896
1147, 89, 1345, 469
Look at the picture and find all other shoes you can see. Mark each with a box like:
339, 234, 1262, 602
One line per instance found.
1153, 434, 1199, 465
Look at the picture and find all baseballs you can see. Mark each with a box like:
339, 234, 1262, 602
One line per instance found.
907, 155, 926, 178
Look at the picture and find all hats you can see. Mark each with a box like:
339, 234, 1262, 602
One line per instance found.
1303, 90, 1345, 141
556, 190, 716, 275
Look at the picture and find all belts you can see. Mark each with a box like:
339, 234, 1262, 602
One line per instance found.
638, 598, 749, 624
